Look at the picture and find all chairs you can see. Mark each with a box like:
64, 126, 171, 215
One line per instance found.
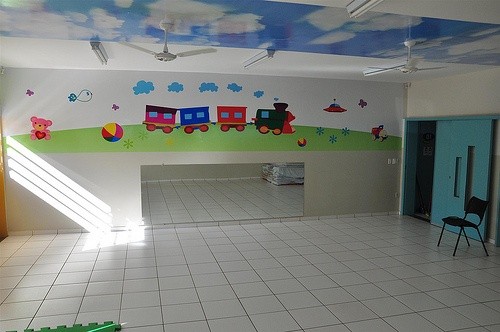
437, 196, 490, 258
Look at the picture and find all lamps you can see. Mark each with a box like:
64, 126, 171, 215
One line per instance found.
90, 42, 109, 65
241, 48, 276, 71
346, 0, 383, 19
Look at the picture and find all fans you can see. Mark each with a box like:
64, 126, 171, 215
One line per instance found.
118, 22, 217, 63
364, 40, 448, 79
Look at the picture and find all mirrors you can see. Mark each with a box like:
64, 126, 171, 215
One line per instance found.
140, 162, 305, 225
